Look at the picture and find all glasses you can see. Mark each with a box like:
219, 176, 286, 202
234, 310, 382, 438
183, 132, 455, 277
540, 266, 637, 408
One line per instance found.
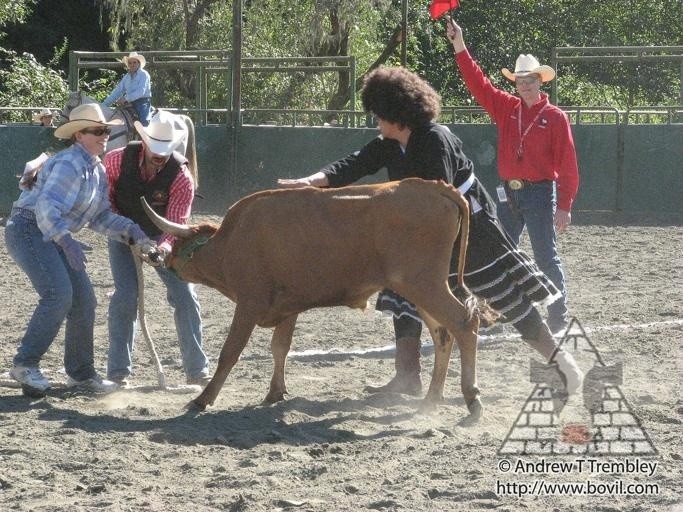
84, 128, 111, 136
514, 76, 539, 86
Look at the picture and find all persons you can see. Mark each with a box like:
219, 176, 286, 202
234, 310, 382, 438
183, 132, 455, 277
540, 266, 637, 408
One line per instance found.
444, 14, 579, 338
275, 60, 583, 420
103, 51, 152, 127
34, 109, 63, 151
100, 109, 216, 389
1, 102, 115, 401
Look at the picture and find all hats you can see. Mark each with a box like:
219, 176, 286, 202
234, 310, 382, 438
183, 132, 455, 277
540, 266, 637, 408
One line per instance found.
134, 110, 188, 157
34, 108, 57, 121
501, 53, 556, 83
123, 51, 146, 70
53, 103, 125, 140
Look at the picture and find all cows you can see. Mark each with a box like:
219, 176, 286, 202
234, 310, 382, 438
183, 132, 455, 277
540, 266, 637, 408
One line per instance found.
529, 358, 624, 446
139, 176, 508, 419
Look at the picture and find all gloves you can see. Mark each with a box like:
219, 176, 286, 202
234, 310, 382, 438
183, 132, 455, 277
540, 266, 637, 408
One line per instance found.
136, 246, 167, 266
127, 223, 148, 244
57, 233, 93, 272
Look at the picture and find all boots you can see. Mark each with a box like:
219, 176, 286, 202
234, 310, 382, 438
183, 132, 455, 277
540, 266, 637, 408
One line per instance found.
517, 320, 584, 395
366, 335, 423, 396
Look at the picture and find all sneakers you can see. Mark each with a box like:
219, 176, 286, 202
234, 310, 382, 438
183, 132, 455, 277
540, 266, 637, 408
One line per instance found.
188, 376, 211, 386
67, 374, 118, 394
9, 362, 51, 392
110, 376, 127, 389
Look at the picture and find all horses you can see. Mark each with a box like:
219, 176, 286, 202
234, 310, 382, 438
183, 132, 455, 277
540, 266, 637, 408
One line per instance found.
58, 89, 198, 192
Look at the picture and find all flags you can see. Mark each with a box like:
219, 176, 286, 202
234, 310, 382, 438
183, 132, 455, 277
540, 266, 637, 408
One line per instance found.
429, 1, 461, 22
